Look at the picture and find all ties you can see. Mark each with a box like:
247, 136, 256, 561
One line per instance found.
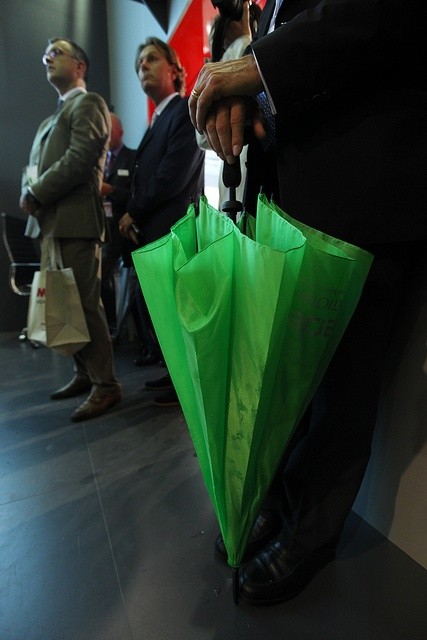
104, 151, 114, 180
150, 113, 158, 130
53, 101, 64, 118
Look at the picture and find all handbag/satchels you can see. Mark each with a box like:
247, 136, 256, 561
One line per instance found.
24, 237, 93, 356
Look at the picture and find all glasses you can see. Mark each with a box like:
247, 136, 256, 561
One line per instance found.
42, 47, 79, 65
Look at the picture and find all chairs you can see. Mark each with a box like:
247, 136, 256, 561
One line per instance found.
2, 212, 41, 350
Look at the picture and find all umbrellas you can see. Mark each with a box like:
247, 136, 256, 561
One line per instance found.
130, 151, 375, 604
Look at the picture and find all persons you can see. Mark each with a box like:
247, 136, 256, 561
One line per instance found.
121, 36, 206, 392
18, 38, 123, 420
187, 0, 427, 604
207, 0, 279, 217
100, 111, 137, 339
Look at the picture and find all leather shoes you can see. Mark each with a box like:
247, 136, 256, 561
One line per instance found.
151, 391, 180, 407
238, 529, 336, 608
71, 391, 122, 422
215, 514, 270, 564
51, 374, 91, 400
145, 374, 173, 390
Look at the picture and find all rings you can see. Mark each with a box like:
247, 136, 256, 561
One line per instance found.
119, 225, 124, 231
190, 88, 199, 98
